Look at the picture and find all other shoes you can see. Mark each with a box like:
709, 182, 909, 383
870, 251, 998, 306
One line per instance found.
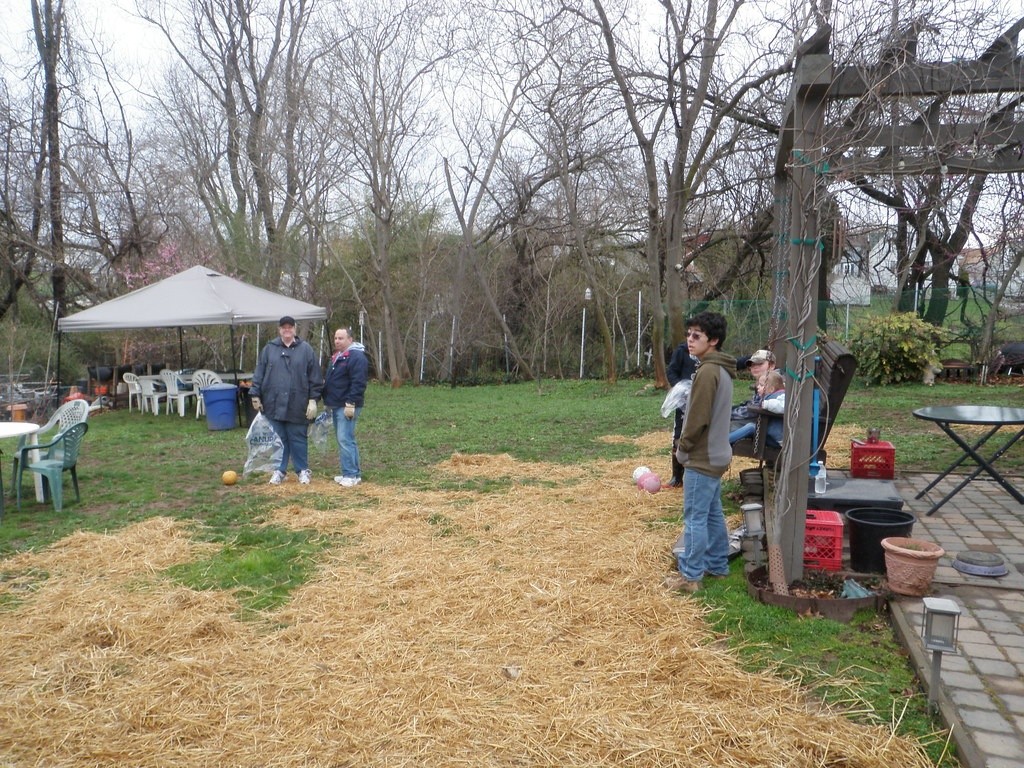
666, 576, 698, 593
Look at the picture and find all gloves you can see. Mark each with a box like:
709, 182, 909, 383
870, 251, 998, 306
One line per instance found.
344, 403, 356, 420
251, 396, 263, 412
305, 399, 318, 419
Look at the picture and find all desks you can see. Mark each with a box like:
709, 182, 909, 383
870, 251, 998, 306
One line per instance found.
0, 421, 44, 503
912, 405, 1024, 516
139, 373, 253, 408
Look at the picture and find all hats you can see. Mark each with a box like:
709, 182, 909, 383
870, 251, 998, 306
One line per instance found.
279, 317, 296, 329
745, 349, 777, 366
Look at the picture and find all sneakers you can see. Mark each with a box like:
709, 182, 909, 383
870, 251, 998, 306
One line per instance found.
268, 468, 285, 483
298, 468, 312, 485
333, 473, 362, 488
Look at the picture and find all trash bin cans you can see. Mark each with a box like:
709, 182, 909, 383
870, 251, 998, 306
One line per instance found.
198, 384, 238, 430
240, 386, 258, 428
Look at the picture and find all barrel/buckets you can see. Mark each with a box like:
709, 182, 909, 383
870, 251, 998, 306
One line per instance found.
846, 507, 917, 574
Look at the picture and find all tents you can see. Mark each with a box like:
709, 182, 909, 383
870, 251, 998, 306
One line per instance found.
56, 265, 332, 425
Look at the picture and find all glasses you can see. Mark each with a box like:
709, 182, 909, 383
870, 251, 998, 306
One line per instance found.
684, 330, 709, 340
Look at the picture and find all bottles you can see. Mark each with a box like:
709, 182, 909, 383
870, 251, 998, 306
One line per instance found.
814, 462, 827, 494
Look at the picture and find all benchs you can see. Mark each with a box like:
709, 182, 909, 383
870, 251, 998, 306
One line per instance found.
732, 341, 859, 488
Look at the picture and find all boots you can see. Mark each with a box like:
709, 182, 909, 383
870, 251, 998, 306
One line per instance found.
667, 447, 685, 488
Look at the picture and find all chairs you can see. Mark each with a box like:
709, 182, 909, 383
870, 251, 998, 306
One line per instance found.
10, 399, 89, 513
123, 369, 223, 419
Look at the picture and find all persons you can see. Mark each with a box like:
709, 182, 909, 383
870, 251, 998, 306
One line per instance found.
63, 386, 85, 404
662, 313, 737, 597
248, 316, 323, 485
321, 327, 368, 487
675, 341, 785, 487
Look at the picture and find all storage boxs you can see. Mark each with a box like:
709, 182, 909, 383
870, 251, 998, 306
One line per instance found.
851, 441, 895, 479
804, 510, 844, 572
